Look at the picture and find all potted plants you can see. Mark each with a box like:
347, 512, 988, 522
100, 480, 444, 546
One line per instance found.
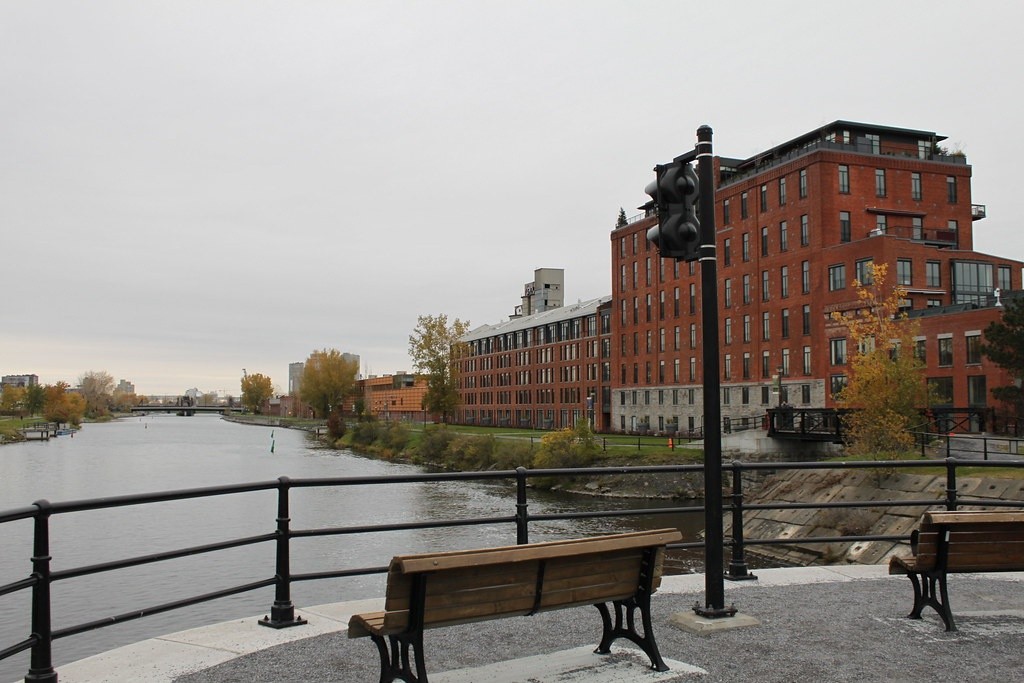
665, 418, 678, 434
637, 417, 649, 436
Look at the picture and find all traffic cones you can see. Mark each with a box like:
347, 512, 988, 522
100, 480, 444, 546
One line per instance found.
668, 438, 673, 447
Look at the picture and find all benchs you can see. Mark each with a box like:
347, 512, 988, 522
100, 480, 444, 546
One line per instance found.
889, 510, 1024, 631
348, 527, 684, 683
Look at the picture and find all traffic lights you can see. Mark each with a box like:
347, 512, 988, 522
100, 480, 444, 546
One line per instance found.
637, 159, 701, 257
